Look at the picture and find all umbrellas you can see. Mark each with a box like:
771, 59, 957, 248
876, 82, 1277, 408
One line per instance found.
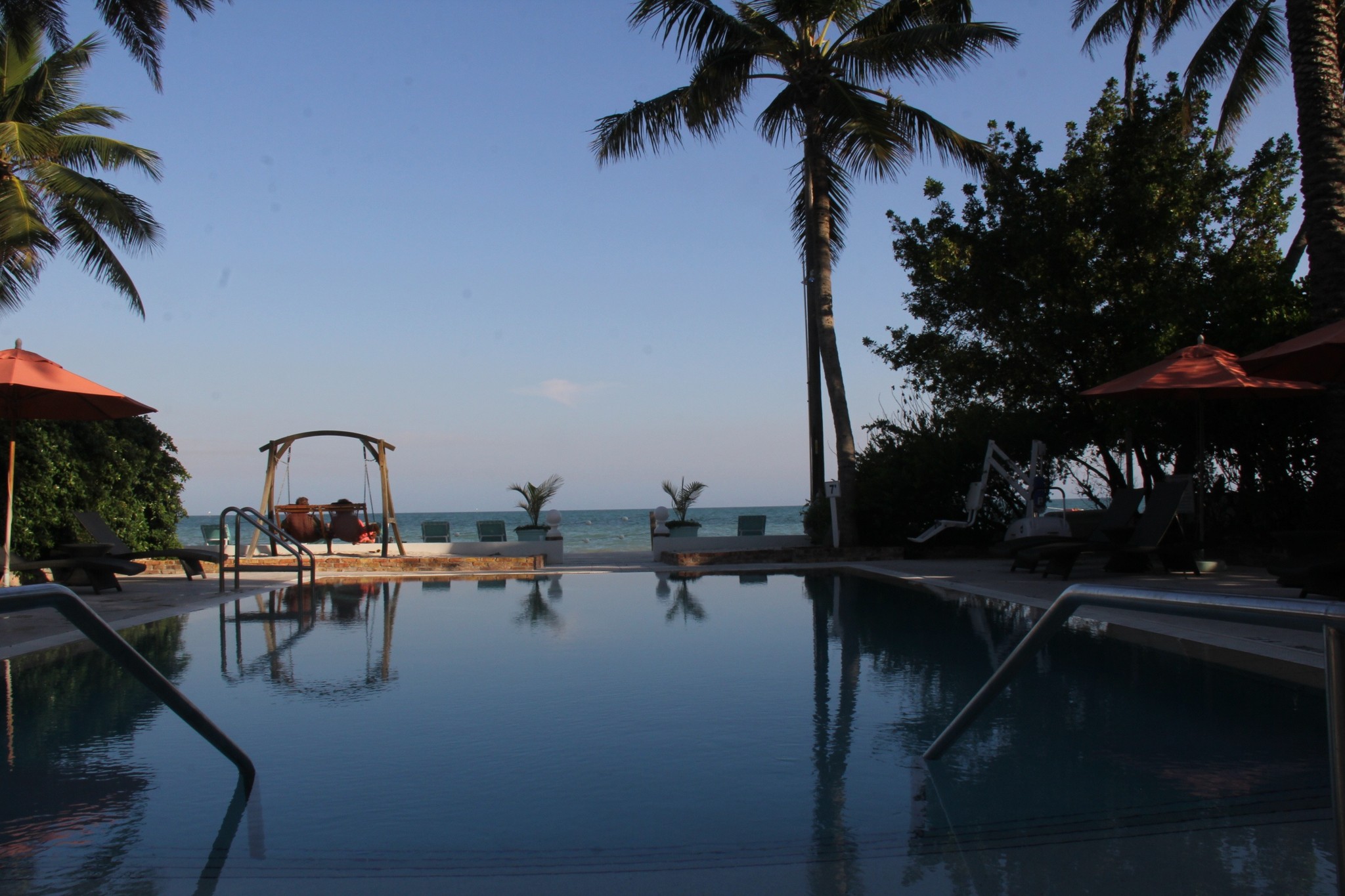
0, 340, 159, 587
1236, 321, 1345, 382
1078, 336, 1319, 573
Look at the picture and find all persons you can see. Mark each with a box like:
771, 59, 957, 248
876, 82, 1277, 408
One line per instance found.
281, 497, 329, 543
327, 499, 378, 544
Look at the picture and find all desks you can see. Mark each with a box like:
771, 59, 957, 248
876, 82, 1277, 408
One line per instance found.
51, 542, 113, 590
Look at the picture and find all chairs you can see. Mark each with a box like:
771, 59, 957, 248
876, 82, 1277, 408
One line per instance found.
1000, 485, 1145, 579
476, 520, 506, 541
1030, 481, 1202, 580
200, 524, 231, 546
74, 510, 229, 580
737, 515, 766, 536
421, 521, 451, 542
0, 546, 146, 593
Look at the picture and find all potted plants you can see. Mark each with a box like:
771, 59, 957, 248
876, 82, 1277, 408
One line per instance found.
512, 479, 557, 541
663, 479, 705, 537
